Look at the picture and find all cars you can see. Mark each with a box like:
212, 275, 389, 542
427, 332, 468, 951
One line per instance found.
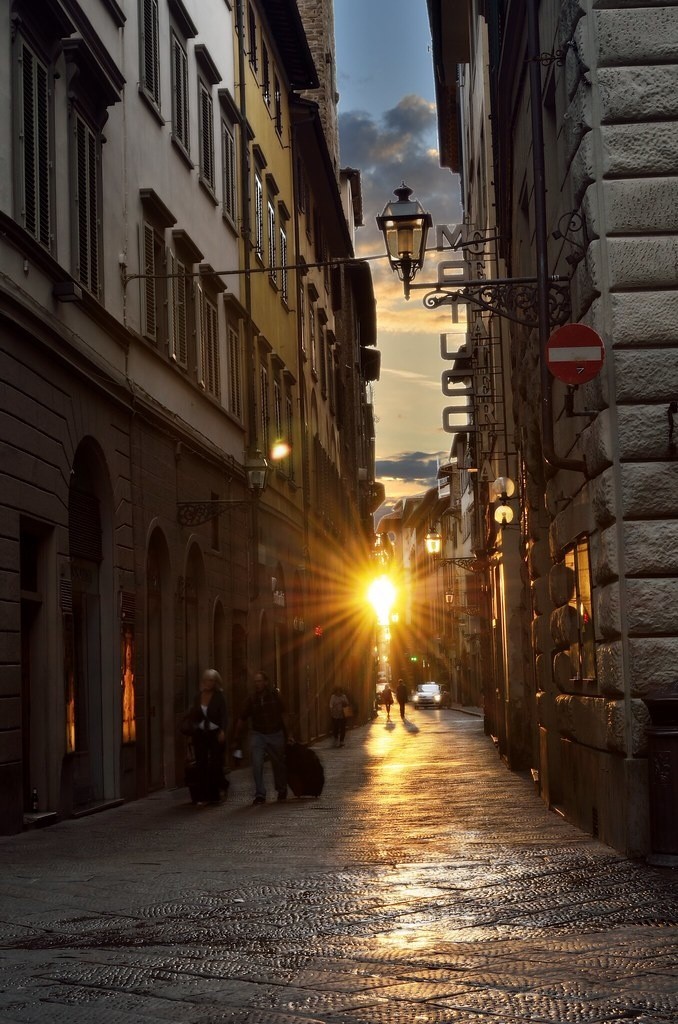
412, 682, 452, 709
377, 683, 390, 703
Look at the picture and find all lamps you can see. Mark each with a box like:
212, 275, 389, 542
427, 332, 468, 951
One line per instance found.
376, 180, 570, 329
424, 527, 486, 572
493, 477, 519, 531
177, 448, 268, 532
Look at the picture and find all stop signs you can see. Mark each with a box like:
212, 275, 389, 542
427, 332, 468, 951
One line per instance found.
543, 321, 605, 384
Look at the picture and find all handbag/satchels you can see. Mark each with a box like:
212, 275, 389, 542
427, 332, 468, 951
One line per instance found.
343, 695, 353, 718
178, 691, 198, 736
272, 743, 289, 784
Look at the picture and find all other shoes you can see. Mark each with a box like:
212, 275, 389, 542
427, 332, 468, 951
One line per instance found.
197, 801, 211, 806
219, 789, 227, 802
253, 797, 265, 804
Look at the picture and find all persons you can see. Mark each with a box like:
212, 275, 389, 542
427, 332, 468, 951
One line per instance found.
177, 669, 230, 797
329, 687, 349, 745
238, 671, 296, 804
382, 679, 408, 719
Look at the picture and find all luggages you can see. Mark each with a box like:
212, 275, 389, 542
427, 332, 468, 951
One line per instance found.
286, 742, 324, 798
